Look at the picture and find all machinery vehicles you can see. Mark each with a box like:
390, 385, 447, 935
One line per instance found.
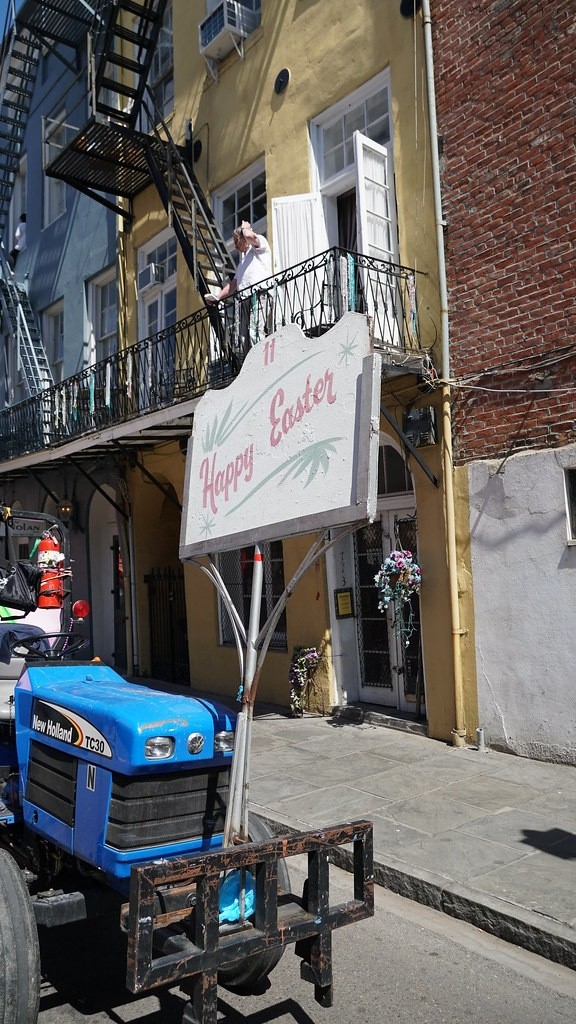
0, 504, 380, 1023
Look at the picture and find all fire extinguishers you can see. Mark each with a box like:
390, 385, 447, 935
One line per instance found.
38, 524, 66, 608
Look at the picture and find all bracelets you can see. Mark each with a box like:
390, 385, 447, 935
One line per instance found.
242, 227, 251, 230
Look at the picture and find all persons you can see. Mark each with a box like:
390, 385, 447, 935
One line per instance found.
203, 220, 274, 353
6, 213, 27, 280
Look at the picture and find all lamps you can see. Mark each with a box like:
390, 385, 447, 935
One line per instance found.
57, 499, 72, 525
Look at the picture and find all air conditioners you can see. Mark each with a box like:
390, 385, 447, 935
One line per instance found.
196, 0, 249, 61
137, 263, 165, 295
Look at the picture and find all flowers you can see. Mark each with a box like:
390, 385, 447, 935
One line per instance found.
375, 549, 422, 652
